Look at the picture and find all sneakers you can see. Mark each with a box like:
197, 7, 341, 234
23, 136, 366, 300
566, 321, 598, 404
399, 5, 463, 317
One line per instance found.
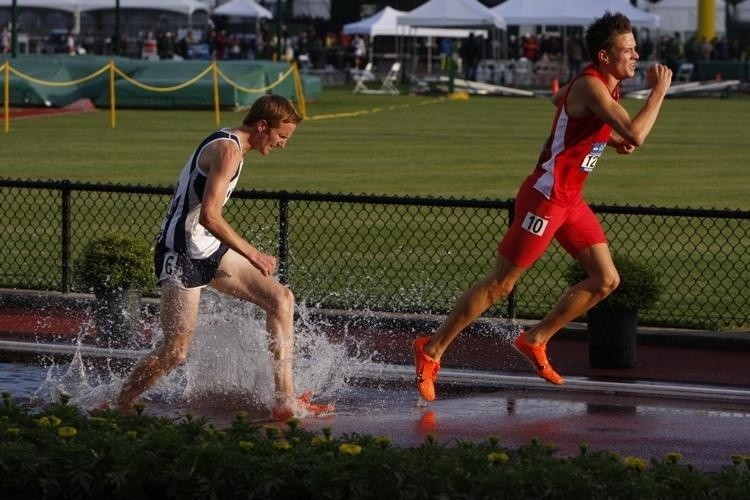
511, 328, 566, 385
413, 337, 441, 402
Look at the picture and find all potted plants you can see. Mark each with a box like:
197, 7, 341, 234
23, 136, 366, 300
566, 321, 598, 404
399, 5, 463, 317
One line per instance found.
565, 247, 666, 368
74, 232, 159, 343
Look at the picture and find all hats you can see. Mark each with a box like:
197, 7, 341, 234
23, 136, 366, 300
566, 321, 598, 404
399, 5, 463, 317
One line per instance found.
268, 401, 335, 420
89, 402, 138, 417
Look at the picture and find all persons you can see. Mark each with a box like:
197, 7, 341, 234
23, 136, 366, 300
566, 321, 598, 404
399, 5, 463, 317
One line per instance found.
412, 10, 673, 402
87, 95, 336, 421
